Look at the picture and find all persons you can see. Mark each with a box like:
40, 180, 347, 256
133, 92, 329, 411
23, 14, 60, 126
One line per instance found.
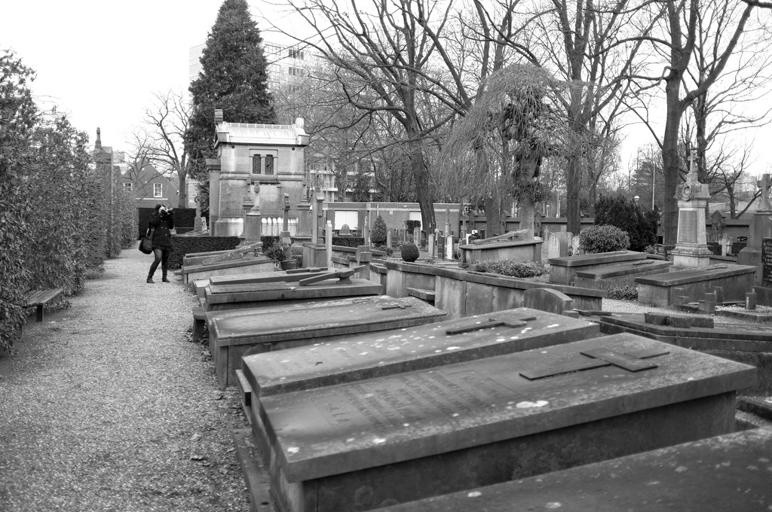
146, 203, 175, 283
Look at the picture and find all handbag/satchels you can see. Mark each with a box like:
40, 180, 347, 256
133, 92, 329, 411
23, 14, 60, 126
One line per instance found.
139, 238, 153, 254
